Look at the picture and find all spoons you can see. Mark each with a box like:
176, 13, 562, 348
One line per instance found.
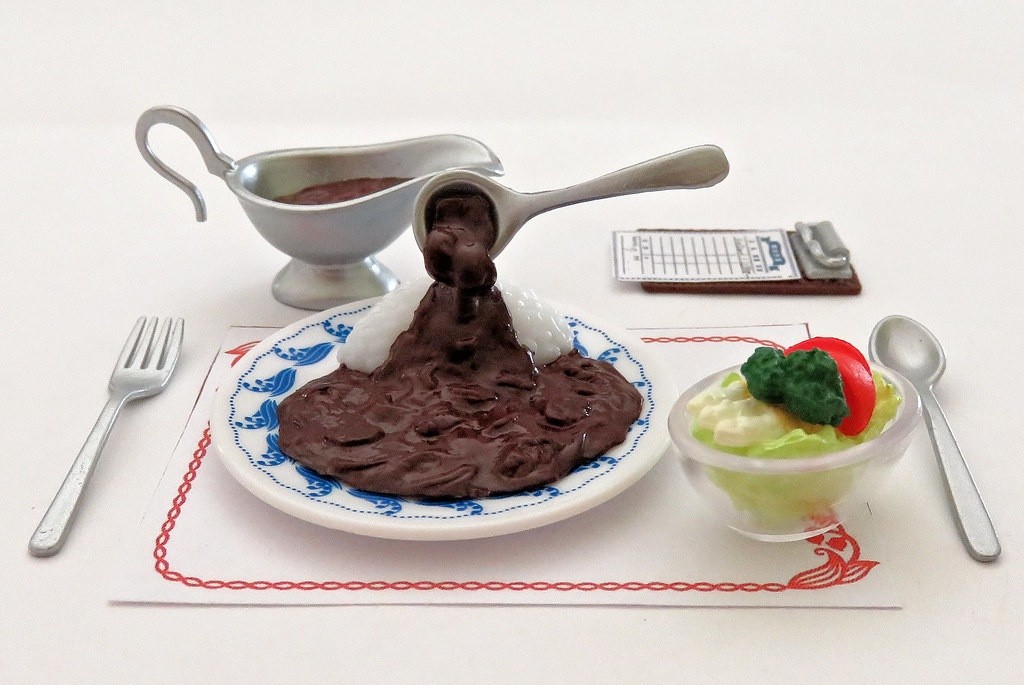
412, 144, 730, 264
868, 316, 1002, 562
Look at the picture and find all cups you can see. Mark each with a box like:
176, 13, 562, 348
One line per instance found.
135, 106, 505, 311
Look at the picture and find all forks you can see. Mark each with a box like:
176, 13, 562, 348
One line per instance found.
28, 317, 192, 558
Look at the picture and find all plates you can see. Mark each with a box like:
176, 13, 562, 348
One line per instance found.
210, 294, 678, 541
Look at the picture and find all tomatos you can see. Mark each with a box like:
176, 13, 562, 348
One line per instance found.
783, 322, 875, 436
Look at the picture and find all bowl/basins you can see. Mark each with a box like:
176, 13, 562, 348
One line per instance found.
668, 358, 921, 542
